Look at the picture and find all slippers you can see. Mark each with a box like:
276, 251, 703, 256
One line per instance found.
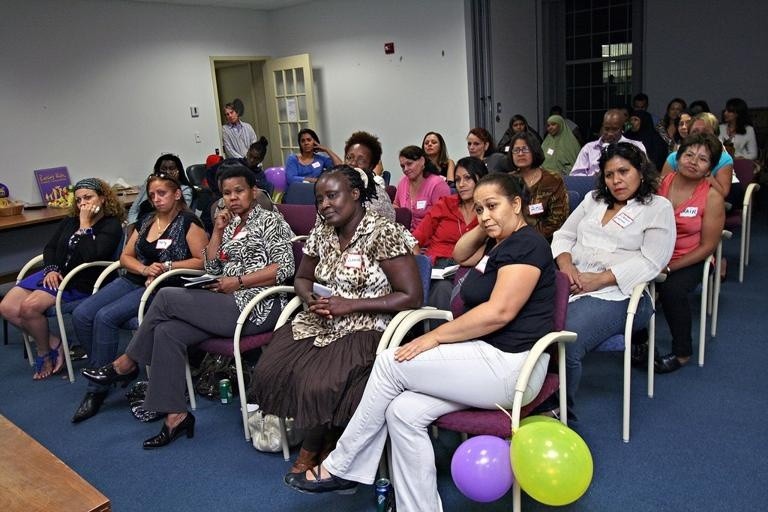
654, 354, 682, 373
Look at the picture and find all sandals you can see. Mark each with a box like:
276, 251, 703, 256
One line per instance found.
33, 353, 52, 381
54, 338, 74, 375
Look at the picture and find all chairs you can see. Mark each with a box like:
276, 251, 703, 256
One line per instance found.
388, 263, 580, 512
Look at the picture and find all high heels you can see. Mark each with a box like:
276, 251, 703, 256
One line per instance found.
71, 391, 108, 423
82, 363, 139, 388
144, 412, 195, 449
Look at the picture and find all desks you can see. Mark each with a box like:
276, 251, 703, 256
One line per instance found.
1, 413, 112, 510
0, 188, 147, 344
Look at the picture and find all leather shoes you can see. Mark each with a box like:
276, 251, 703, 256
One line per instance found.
284, 464, 359, 496
286, 449, 316, 473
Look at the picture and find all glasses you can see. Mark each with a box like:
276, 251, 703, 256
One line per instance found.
149, 173, 181, 188
602, 142, 641, 156
512, 146, 532, 153
345, 154, 368, 165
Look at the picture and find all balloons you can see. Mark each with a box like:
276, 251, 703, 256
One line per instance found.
508, 423, 594, 507
449, 434, 516, 503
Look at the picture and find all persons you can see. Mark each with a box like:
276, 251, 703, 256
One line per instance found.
248, 164, 426, 476
0, 176, 128, 385
78, 162, 297, 449
69, 169, 211, 426
603, 74, 617, 92
126, 89, 766, 429
282, 172, 560, 512
